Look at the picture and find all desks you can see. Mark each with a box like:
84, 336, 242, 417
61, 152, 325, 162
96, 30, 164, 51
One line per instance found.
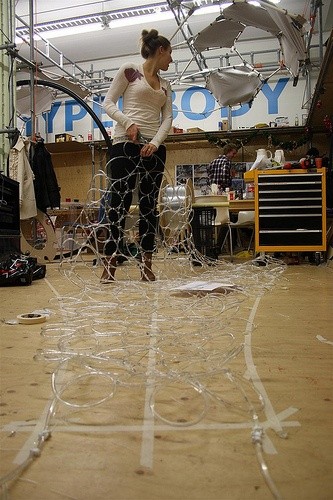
228, 200, 256, 252
47, 210, 101, 264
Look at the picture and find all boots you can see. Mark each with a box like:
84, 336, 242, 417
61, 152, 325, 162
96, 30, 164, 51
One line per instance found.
99, 258, 117, 284
141, 254, 155, 281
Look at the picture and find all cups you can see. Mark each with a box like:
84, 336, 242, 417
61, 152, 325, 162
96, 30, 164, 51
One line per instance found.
315, 157, 322, 168
304, 157, 316, 168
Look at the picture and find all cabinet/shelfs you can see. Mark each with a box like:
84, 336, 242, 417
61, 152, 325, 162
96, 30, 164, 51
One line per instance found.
252, 167, 327, 262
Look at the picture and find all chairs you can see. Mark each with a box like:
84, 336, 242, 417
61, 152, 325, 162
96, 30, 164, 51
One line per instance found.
58, 188, 111, 265
193, 194, 256, 259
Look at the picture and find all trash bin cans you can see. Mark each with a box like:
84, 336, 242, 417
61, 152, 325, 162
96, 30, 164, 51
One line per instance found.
189, 207, 219, 266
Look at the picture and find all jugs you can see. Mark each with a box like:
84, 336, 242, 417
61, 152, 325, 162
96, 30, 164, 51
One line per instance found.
255, 149, 272, 168
274, 150, 285, 165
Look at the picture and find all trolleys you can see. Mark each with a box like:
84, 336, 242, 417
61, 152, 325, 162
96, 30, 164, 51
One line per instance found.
243, 166, 328, 264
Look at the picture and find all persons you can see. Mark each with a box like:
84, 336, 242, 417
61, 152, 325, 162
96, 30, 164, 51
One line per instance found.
207, 143, 238, 255
98, 28, 174, 284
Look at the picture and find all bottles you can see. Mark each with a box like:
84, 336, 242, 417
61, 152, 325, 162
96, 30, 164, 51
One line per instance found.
106, 126, 112, 140
72, 130, 92, 142
211, 177, 254, 201
